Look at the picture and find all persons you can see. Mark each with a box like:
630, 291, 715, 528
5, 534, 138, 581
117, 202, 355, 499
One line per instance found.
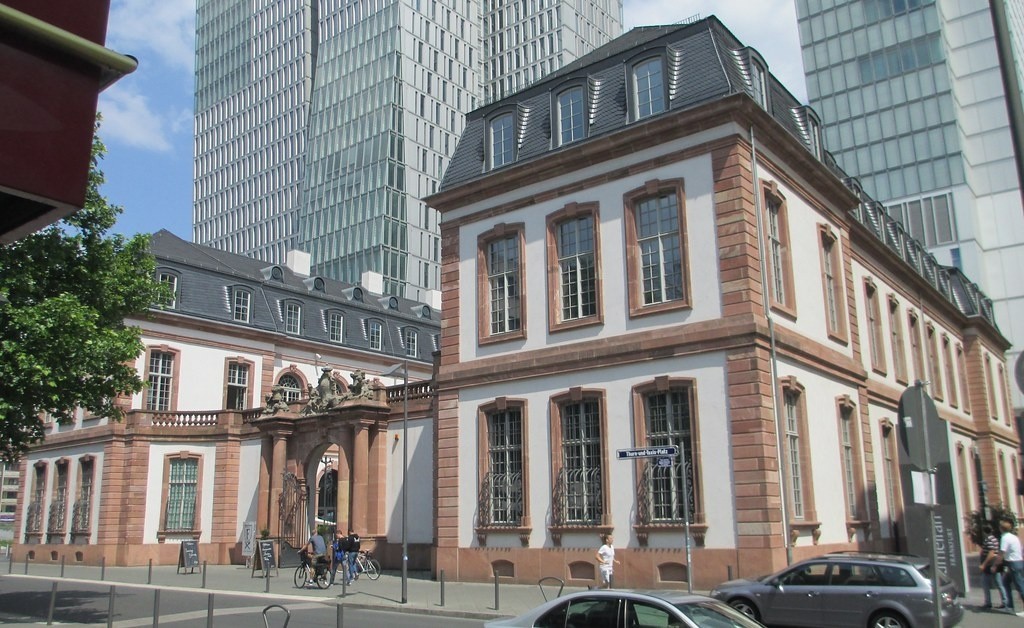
997, 521, 1024, 614
346, 529, 360, 579
593, 534, 620, 589
325, 530, 351, 588
297, 529, 327, 584
976, 525, 1007, 610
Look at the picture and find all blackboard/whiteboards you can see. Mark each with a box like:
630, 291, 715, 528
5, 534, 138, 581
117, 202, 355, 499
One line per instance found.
253, 540, 277, 570
178, 541, 201, 567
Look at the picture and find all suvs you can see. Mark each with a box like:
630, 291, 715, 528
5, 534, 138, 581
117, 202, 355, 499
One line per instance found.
710, 548, 964, 628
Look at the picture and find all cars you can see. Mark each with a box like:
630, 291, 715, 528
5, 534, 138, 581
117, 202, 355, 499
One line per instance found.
482, 586, 773, 628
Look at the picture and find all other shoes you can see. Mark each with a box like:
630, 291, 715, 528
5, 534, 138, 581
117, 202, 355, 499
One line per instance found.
982, 604, 992, 609
347, 582, 351, 585
999, 603, 1005, 608
351, 578, 354, 582
1002, 605, 1016, 615
305, 580, 313, 585
330, 584, 334, 587
355, 574, 359, 580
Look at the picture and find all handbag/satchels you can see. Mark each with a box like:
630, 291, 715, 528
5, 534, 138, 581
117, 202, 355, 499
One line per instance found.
316, 563, 327, 575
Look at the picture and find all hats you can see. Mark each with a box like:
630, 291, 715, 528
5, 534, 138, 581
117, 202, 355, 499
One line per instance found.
999, 519, 1012, 531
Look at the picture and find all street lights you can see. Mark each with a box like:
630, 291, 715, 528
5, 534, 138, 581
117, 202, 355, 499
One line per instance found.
378, 359, 409, 604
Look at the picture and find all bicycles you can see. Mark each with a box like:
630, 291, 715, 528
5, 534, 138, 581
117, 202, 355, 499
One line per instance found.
294, 550, 333, 590
355, 549, 383, 581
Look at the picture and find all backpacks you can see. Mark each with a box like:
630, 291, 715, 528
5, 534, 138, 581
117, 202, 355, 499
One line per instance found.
338, 537, 352, 551
